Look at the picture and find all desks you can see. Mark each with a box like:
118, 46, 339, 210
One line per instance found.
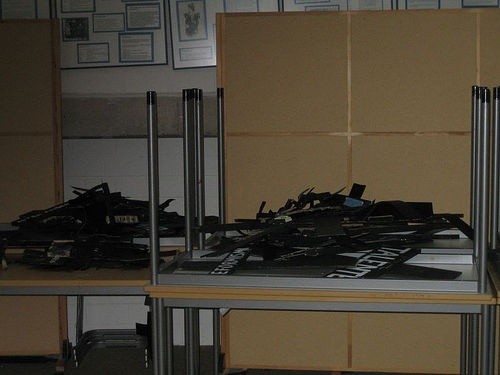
0, 86, 500, 375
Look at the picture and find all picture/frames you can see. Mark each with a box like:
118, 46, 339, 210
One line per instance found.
283, 1, 498, 11
169, 0, 280, 71
48, 0, 168, 69
